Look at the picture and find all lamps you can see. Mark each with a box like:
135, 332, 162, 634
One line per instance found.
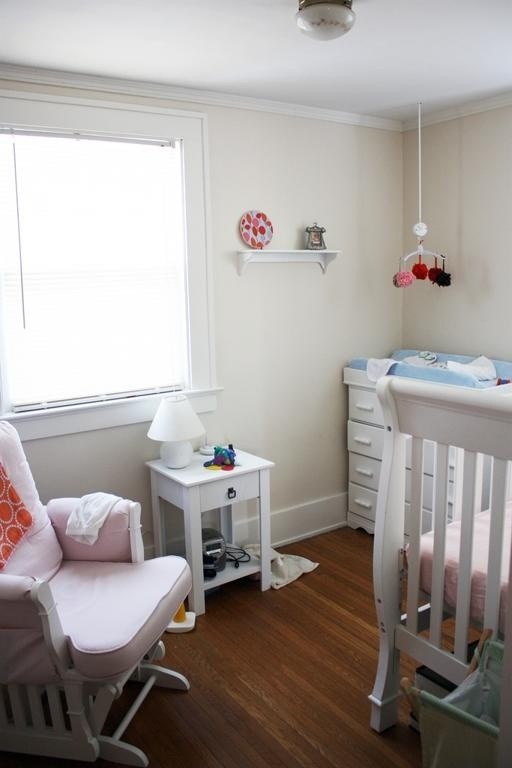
294, 0, 358, 39
144, 395, 206, 468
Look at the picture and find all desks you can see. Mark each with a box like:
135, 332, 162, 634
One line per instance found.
144, 445, 276, 619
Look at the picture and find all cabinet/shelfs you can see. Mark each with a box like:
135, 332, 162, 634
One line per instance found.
340, 366, 490, 556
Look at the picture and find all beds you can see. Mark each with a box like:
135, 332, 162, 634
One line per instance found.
369, 375, 512, 730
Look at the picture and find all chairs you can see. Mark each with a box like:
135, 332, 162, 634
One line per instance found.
0, 420, 193, 767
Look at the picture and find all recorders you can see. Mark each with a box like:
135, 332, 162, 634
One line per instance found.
202, 528, 226, 572
